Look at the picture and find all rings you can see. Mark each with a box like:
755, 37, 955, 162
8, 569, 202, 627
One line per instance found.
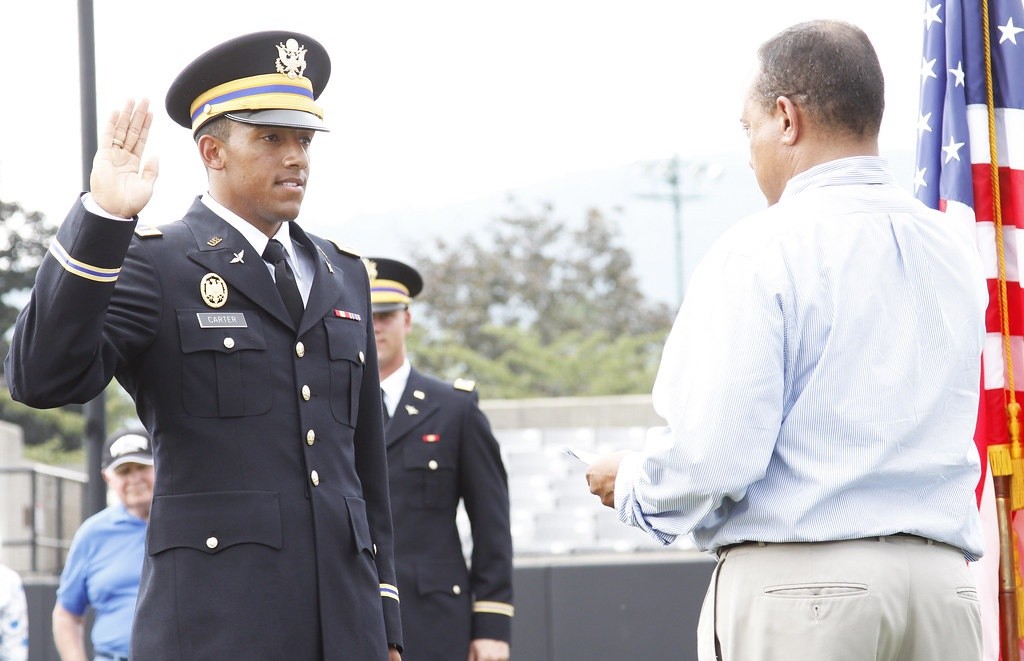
112, 139, 124, 147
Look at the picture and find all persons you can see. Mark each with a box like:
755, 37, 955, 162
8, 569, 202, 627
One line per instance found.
50, 428, 155, 661
361, 257, 513, 661
585, 18, 992, 661
4, 30, 404, 661
0, 565, 29, 661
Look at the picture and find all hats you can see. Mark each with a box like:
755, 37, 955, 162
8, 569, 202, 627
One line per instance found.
100, 427, 155, 471
161, 29, 335, 134
362, 255, 424, 315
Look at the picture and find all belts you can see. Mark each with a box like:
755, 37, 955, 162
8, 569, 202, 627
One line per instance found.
719, 530, 927, 551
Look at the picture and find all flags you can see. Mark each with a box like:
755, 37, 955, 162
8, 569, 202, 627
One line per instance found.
914, 0, 1024, 661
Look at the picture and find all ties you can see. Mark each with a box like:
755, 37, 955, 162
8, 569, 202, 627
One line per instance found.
262, 238, 308, 328
380, 387, 392, 429
